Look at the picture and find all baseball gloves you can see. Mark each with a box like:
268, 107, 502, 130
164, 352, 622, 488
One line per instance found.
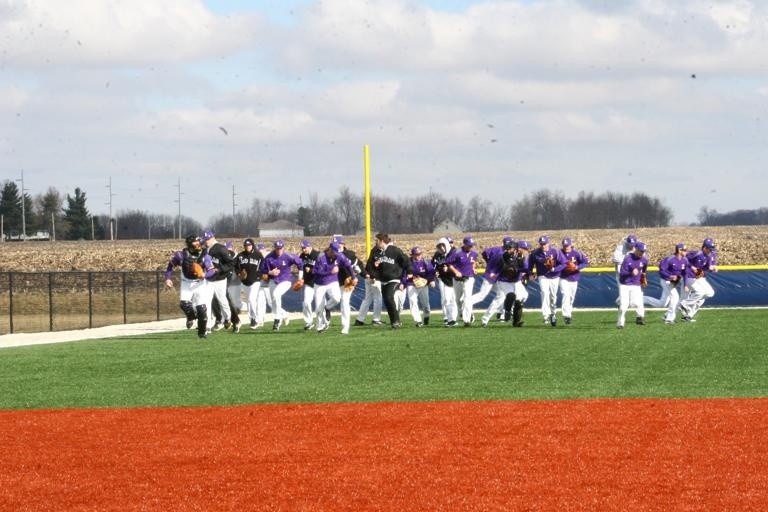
412, 276, 428, 288
192, 262, 203, 278
544, 257, 553, 270
240, 269, 248, 281
293, 280, 304, 291
565, 261, 576, 273
344, 277, 355, 291
697, 268, 704, 278
641, 273, 647, 288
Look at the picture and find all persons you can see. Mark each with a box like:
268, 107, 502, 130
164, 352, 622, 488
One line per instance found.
710, 246, 718, 274
528, 236, 568, 326
164, 233, 216, 337
204, 229, 415, 337
559, 238, 588, 324
678, 239, 717, 323
611, 236, 638, 307
617, 243, 650, 327
393, 237, 535, 329
643, 242, 690, 323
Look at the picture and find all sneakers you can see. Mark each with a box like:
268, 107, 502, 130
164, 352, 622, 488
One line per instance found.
661, 315, 679, 325
340, 326, 349, 334
371, 320, 387, 326
616, 322, 626, 330
440, 310, 573, 328
391, 321, 403, 330
303, 323, 315, 330
184, 312, 292, 339
635, 316, 648, 326
317, 305, 332, 333
680, 314, 697, 323
677, 303, 690, 318
423, 316, 430, 325
353, 318, 365, 326
416, 321, 424, 328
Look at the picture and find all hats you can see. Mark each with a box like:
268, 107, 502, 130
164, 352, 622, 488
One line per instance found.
273, 239, 284, 250
538, 234, 550, 246
702, 236, 717, 250
501, 235, 533, 250
625, 234, 639, 247
636, 241, 648, 253
185, 229, 266, 253
410, 246, 424, 256
300, 238, 311, 250
676, 241, 689, 252
446, 236, 455, 244
561, 236, 573, 248
463, 236, 478, 247
329, 242, 343, 256
332, 234, 346, 244
